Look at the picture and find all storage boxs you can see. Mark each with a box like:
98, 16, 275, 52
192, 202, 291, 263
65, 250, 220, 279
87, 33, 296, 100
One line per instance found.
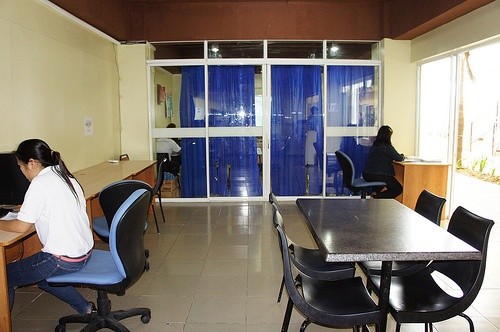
160, 178, 180, 198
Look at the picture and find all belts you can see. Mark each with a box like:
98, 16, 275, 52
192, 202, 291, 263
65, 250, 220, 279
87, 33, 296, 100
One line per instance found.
52, 249, 93, 262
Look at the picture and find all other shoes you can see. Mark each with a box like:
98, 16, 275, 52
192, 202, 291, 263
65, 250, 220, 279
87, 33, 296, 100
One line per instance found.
85, 301, 97, 314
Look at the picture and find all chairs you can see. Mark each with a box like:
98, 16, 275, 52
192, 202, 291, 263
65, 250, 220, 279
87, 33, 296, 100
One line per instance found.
156, 153, 169, 173
335, 150, 386, 199
269, 190, 495, 332
44, 189, 151, 332
91, 180, 153, 259
150, 158, 167, 234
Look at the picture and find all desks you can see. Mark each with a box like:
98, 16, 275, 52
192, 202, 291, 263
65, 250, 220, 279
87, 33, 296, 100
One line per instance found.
392, 159, 452, 220
296, 198, 482, 332
0, 160, 157, 332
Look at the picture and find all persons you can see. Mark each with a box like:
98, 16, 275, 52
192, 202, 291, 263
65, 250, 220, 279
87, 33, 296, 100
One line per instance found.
362, 126, 406, 199
155, 123, 181, 175
339, 124, 372, 177
308, 106, 323, 148
5, 139, 98, 314
